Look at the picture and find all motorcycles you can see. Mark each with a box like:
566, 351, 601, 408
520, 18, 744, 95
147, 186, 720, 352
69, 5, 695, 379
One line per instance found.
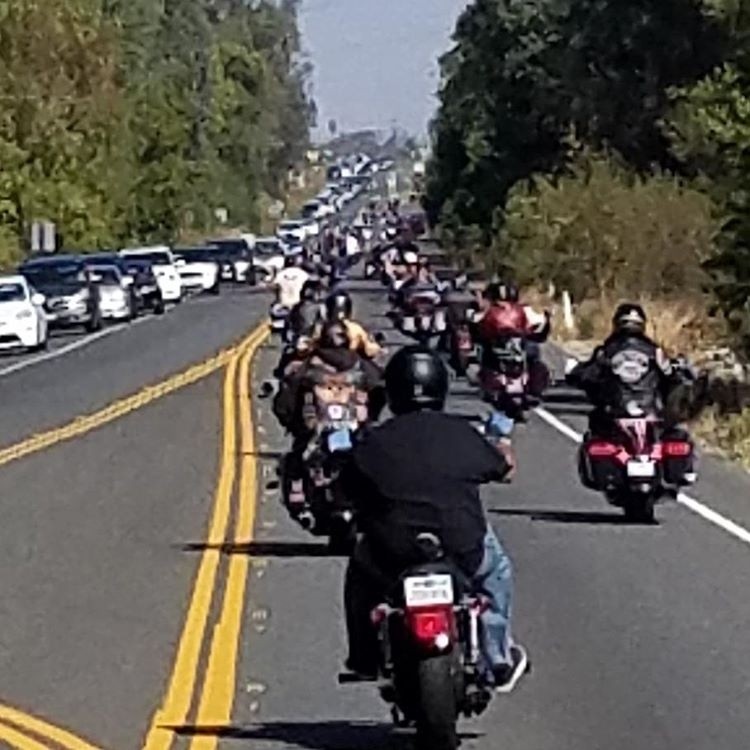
328, 415, 532, 750
368, 215, 550, 422
263, 226, 390, 553
564, 354, 698, 523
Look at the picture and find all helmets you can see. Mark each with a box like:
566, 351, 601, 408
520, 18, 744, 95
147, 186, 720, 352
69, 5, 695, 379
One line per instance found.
300, 279, 328, 301
488, 282, 519, 303
326, 289, 352, 316
379, 344, 448, 411
612, 304, 647, 330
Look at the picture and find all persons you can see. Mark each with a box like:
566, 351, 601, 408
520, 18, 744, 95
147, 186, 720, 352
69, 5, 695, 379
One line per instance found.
565, 304, 698, 492
328, 348, 525, 694
266, 214, 550, 513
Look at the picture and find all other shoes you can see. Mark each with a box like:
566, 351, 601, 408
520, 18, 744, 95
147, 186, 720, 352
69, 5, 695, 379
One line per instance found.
492, 645, 527, 693
344, 657, 378, 682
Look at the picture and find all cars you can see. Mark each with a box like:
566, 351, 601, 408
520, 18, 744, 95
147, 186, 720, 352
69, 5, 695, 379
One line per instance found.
0, 238, 283, 352
